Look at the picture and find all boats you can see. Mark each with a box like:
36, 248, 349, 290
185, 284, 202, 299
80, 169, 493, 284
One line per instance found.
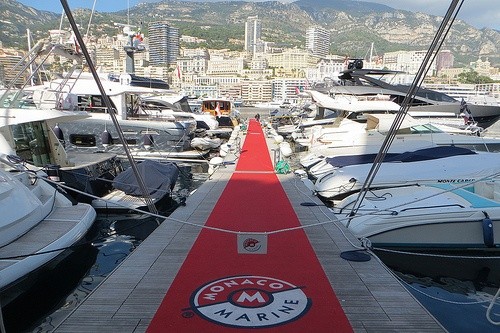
0, 0, 238, 325
266, 57, 500, 290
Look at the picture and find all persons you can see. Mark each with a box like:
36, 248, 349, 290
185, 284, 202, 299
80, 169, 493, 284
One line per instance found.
215, 102, 221, 127
255, 112, 260, 123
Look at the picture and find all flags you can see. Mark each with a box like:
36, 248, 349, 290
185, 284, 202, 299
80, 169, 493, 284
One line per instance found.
174, 66, 182, 80
